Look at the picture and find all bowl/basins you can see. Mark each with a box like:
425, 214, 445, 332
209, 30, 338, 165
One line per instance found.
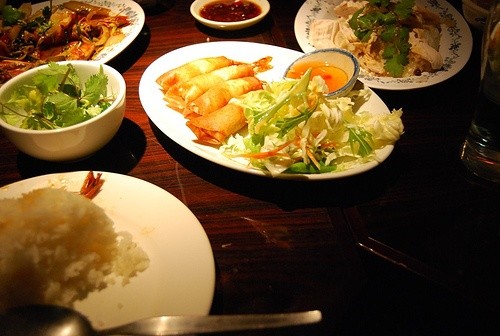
462, 0, 489, 28
0, 61, 127, 160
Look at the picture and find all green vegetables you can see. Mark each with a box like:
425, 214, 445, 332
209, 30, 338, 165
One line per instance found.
348, 0, 416, 77
0, 61, 116, 131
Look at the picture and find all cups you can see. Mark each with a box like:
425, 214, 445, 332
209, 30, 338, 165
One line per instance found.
458, 0, 500, 183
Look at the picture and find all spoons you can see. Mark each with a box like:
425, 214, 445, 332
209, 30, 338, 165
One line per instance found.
0, 303, 322, 336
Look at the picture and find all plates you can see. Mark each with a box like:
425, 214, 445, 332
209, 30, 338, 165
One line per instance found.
138, 40, 395, 180
282, 47, 360, 98
0, 170, 216, 336
294, 0, 473, 90
31, 0, 145, 63
190, 0, 270, 30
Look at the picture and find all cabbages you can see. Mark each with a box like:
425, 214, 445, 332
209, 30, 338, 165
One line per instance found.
220, 67, 405, 177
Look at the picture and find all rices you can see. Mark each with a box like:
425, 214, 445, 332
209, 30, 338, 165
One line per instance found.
0, 188, 149, 311
